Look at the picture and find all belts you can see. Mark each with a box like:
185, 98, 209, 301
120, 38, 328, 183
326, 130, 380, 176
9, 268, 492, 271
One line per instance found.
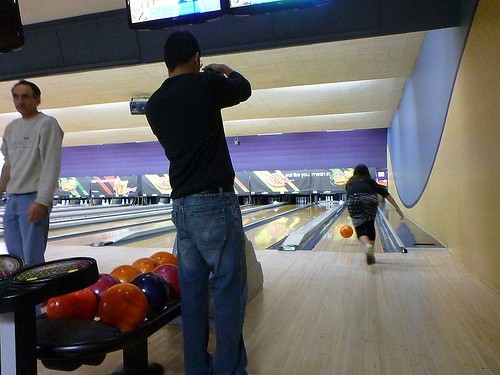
199, 186, 234, 194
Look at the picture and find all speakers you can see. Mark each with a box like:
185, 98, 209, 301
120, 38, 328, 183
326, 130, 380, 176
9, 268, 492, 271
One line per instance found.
130, 102, 148, 115
0, 0, 26, 53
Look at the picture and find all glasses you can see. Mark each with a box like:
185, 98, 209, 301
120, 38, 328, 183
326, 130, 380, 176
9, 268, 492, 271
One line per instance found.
198, 57, 203, 68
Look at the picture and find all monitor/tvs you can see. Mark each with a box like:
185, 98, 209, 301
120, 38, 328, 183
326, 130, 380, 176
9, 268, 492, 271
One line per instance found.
125, 0, 316, 29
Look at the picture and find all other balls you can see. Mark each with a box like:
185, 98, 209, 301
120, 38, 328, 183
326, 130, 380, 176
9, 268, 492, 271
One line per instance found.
43, 250, 181, 332
340, 225, 353, 238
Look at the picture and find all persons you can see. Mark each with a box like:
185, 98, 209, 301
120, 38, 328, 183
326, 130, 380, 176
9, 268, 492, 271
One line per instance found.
146, 31, 252, 375
0, 81, 64, 316
346, 164, 403, 264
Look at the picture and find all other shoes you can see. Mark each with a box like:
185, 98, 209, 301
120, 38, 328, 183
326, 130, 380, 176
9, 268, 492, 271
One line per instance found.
365, 244, 374, 265
